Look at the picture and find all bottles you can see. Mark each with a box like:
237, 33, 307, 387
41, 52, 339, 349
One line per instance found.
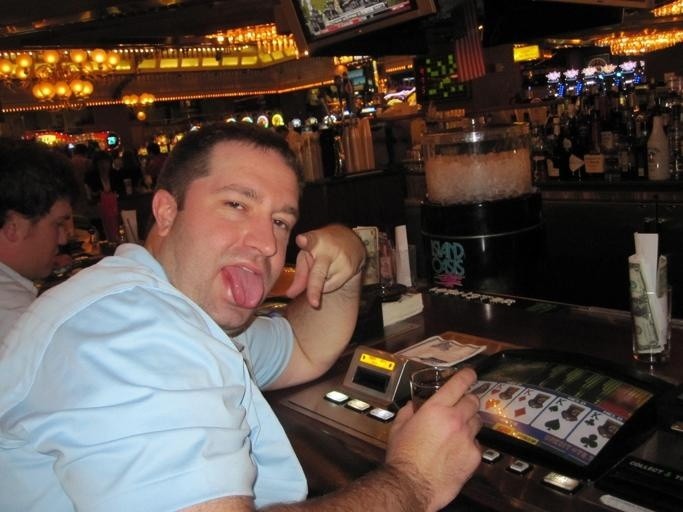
522, 77, 682, 187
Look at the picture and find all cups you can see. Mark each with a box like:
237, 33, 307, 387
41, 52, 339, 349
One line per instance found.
390, 244, 421, 287
628, 288, 674, 365
407, 365, 457, 413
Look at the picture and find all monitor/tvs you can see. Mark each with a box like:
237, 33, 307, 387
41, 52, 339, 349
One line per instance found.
106, 134, 121, 149
280, 0, 436, 53
404, 344, 678, 473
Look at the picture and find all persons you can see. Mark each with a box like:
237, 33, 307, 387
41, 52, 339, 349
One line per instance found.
54, 141, 163, 242
0, 134, 78, 344
0, 121, 483, 512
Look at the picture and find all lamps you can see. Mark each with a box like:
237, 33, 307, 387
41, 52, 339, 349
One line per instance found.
0, 48, 121, 113
122, 92, 155, 120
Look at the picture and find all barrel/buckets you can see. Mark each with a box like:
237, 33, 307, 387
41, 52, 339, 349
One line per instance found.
421, 193, 541, 293
